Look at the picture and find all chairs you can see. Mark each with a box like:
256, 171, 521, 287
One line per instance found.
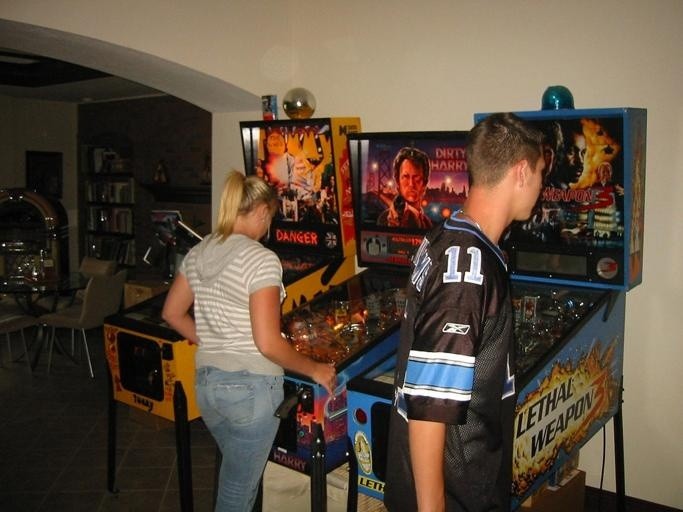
1, 255, 129, 379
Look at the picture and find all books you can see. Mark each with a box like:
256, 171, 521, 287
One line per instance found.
85, 146, 136, 267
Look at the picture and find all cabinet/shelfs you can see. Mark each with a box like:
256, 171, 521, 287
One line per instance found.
76, 132, 158, 281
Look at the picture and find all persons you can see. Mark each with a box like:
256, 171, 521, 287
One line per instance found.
379, 112, 546, 511
374, 147, 435, 230
158, 167, 338, 512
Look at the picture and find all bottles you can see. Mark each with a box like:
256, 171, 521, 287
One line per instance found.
40, 249, 57, 280
262, 95, 277, 120
